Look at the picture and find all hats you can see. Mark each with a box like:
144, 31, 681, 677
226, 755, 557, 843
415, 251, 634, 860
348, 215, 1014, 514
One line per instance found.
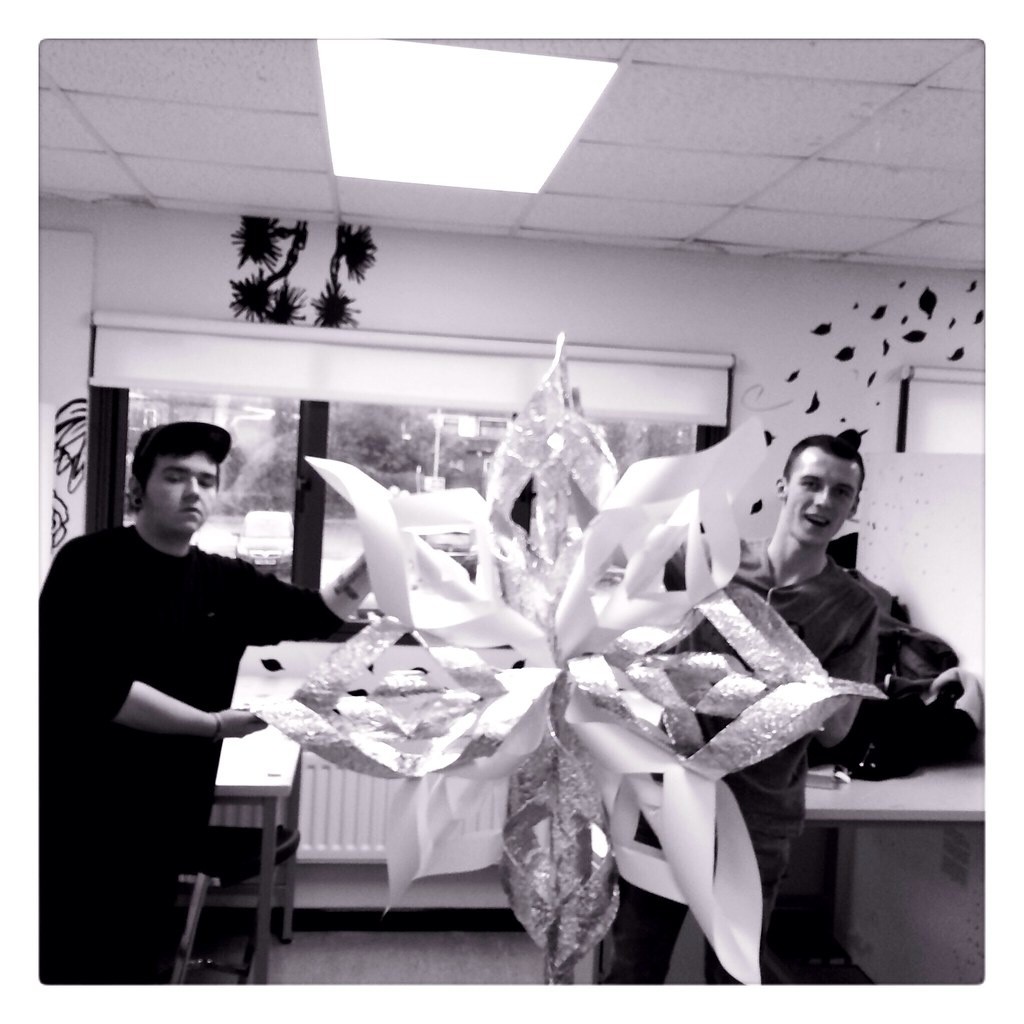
132, 422, 231, 478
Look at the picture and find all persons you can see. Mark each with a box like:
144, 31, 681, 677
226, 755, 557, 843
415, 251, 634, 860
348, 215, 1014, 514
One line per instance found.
591, 431, 882, 985
39, 420, 412, 985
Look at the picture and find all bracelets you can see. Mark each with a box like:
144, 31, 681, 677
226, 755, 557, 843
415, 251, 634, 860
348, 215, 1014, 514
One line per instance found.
212, 712, 221, 739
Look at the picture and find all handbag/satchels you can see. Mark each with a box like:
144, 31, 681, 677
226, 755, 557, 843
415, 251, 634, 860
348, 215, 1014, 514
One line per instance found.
840, 680, 978, 781
